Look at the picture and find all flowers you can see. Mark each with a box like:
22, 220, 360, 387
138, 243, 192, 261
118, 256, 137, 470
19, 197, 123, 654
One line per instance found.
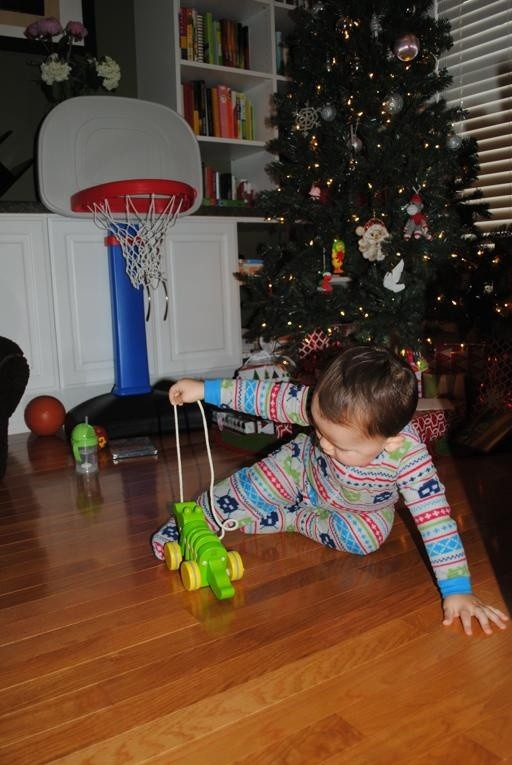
23, 15, 122, 108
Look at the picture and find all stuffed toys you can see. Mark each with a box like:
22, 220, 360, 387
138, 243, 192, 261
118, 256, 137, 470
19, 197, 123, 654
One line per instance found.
355, 217, 390, 263
399, 192, 430, 243
93, 425, 109, 452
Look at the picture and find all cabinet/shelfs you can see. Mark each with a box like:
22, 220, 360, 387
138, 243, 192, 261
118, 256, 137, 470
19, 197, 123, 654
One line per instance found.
133, 0, 299, 215
0, 211, 300, 437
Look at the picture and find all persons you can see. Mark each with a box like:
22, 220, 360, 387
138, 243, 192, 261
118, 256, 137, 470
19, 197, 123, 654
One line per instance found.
151, 343, 509, 638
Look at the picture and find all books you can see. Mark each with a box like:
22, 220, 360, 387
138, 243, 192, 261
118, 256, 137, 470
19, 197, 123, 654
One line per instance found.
181, 77, 256, 141
177, 4, 250, 70
274, 29, 289, 77
199, 162, 251, 206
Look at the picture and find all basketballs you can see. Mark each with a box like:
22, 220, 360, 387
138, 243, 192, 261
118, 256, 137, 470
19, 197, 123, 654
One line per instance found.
24, 395, 65, 435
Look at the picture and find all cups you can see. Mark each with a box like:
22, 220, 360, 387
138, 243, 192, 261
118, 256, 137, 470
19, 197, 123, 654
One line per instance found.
70, 423, 100, 475
